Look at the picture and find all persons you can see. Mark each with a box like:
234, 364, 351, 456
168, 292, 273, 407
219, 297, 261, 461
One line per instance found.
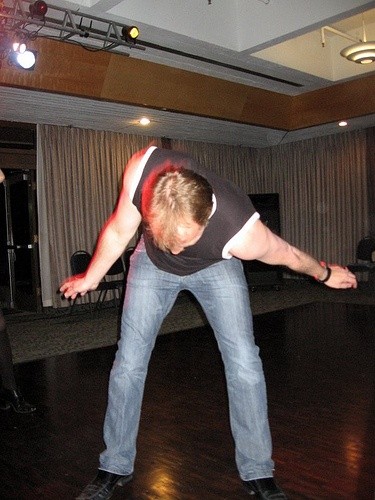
0, 312, 37, 414
59, 144, 358, 500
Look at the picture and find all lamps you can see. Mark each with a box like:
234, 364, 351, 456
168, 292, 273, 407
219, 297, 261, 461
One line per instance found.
29, 1, 48, 16
122, 25, 139, 38
340, 13, 375, 64
10, 50, 36, 69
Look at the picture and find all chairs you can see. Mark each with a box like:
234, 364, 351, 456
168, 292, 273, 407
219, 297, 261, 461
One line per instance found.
70, 247, 135, 313
345, 236, 375, 281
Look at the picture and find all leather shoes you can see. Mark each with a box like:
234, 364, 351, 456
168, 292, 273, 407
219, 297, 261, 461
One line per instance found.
243, 477, 288, 500
74, 468, 134, 500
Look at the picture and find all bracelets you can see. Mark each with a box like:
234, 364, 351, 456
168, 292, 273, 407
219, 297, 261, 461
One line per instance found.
318, 266, 331, 283
